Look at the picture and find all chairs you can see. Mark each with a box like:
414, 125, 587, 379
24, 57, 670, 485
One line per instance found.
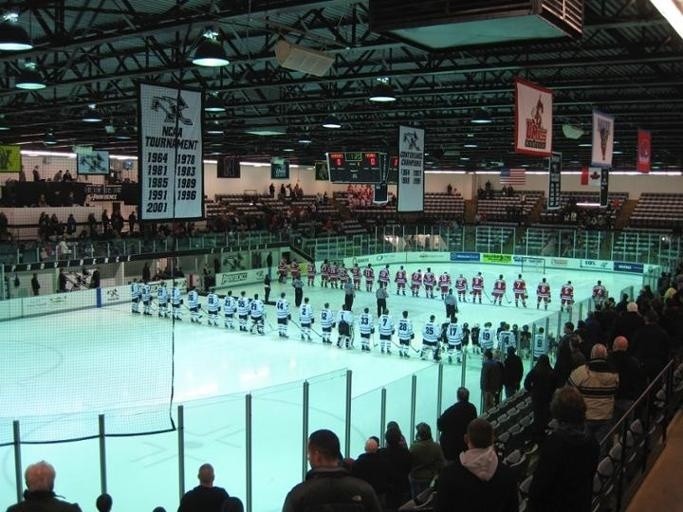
478, 370, 674, 510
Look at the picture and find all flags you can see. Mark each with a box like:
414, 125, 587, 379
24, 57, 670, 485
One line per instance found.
499, 167, 527, 186
580, 165, 603, 186
74, 149, 110, 176
0, 145, 21, 174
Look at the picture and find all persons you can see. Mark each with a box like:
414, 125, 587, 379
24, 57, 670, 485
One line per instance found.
6, 461, 82, 511
559, 195, 618, 250
237, 290, 248, 332
206, 288, 220, 327
523, 267, 682, 443
186, 285, 201, 324
0, 165, 281, 296
129, 278, 141, 314
177, 463, 229, 512
263, 273, 272, 305
156, 281, 170, 318
445, 177, 515, 198
223, 290, 237, 329
139, 280, 152, 316
278, 260, 607, 312
221, 496, 245, 512
290, 277, 558, 362
96, 493, 112, 511
282, 347, 600, 511
279, 183, 374, 244
600, 124, 610, 160
248, 294, 265, 335
317, 164, 328, 180
167, 281, 183, 321
415, 205, 523, 240
276, 292, 291, 338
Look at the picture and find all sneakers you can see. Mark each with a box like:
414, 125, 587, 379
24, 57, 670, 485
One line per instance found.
301, 334, 312, 341
174, 316, 182, 320
224, 324, 264, 334
516, 301, 570, 312
279, 331, 289, 338
323, 338, 371, 351
307, 282, 343, 290
418, 356, 462, 364
131, 309, 168, 318
191, 318, 219, 326
397, 291, 444, 299
473, 349, 480, 354
458, 297, 502, 306
380, 347, 410, 358
354, 286, 372, 291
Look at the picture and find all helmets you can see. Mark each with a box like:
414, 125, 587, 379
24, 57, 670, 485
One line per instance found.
513, 324, 528, 331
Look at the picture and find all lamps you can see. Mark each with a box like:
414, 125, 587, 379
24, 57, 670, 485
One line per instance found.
191, 1, 230, 70
282, 134, 312, 155
322, 115, 343, 129
370, 71, 397, 105
0, 3, 50, 92
0, 106, 133, 148
459, 108, 493, 160
202, 94, 228, 158
